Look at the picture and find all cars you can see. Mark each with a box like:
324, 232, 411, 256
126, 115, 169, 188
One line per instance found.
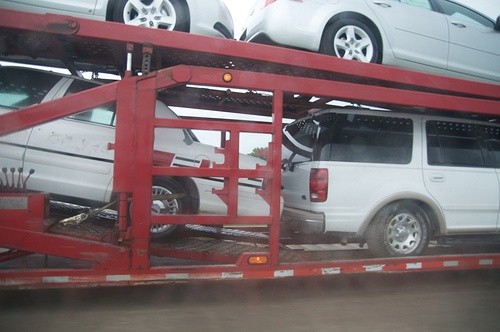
1, 62, 284, 243
0, 1, 498, 85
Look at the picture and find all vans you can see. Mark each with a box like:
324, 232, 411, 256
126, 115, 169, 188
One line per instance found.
277, 106, 500, 257
